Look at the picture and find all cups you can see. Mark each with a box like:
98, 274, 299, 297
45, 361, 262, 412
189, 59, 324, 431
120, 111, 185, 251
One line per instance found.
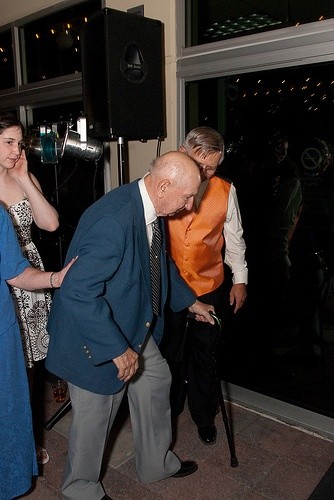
51, 380, 68, 403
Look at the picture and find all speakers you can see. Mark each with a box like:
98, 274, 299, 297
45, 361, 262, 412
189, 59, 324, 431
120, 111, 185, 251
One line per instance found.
80, 8, 167, 140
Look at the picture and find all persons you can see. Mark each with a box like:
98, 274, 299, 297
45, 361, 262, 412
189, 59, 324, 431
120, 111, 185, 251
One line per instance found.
0, 113, 81, 500
166, 126, 250, 445
44, 151, 199, 500
247, 138, 334, 368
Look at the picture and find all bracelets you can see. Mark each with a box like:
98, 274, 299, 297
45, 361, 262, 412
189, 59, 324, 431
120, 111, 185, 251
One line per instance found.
49, 272, 57, 288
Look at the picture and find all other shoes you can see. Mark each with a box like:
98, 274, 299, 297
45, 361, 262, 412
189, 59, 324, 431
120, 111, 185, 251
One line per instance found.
34, 445, 50, 465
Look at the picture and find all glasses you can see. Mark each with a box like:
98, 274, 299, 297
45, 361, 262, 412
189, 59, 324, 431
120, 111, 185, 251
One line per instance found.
198, 165, 218, 176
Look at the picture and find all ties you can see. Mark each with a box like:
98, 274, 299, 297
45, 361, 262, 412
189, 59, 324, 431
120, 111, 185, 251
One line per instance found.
149, 218, 164, 316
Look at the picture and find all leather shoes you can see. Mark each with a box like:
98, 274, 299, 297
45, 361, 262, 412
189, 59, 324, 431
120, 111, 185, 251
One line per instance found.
193, 414, 217, 444
170, 460, 197, 477
